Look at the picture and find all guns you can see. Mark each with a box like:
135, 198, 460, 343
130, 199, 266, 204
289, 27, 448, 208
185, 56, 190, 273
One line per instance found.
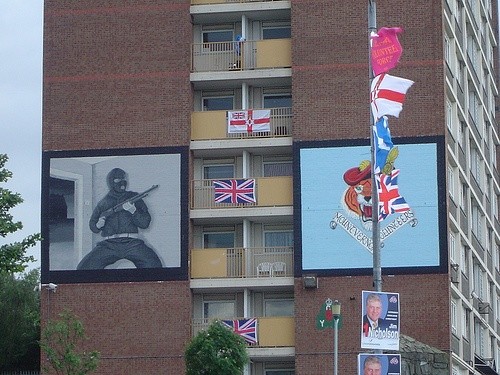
100, 185, 159, 218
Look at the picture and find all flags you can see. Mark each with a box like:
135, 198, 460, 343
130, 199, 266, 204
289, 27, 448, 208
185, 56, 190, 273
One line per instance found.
369, 25, 404, 77
227, 109, 271, 133
376, 167, 410, 222
213, 178, 257, 204
221, 317, 258, 343
370, 72, 415, 124
371, 115, 395, 170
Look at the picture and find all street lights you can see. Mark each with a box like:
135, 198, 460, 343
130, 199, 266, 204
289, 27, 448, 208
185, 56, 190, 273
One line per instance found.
332, 301, 341, 375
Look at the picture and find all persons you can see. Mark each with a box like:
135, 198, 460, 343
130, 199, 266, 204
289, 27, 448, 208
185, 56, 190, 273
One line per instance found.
362, 293, 390, 338
75, 167, 163, 268
363, 356, 381, 375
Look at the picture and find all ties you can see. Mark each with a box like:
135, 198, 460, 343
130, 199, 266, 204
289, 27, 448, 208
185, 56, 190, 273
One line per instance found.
373, 322, 377, 328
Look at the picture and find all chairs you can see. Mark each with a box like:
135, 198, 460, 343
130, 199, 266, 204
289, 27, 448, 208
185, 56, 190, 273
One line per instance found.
256, 262, 273, 278
270, 262, 287, 278
